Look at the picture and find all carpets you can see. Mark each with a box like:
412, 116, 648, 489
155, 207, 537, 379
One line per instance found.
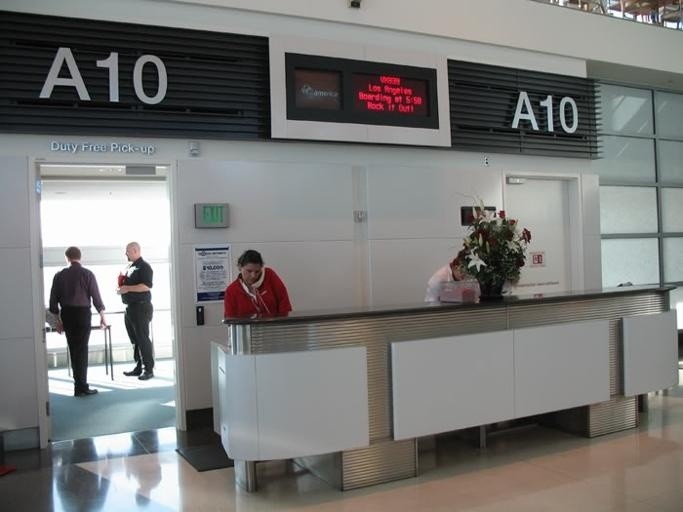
173, 442, 233, 472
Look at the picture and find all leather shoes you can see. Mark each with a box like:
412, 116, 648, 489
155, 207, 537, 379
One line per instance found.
124, 368, 142, 376
138, 369, 153, 379
75, 389, 97, 396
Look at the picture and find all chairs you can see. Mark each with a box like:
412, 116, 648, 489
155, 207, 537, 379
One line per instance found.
547, 0, 683, 29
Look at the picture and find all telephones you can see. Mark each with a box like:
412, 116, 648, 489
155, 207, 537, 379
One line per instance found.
251, 266, 265, 289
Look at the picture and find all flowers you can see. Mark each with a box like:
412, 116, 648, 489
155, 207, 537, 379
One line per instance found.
453, 191, 532, 288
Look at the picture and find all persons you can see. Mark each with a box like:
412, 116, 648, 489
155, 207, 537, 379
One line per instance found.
49, 247, 110, 398
115, 241, 155, 380
423, 250, 479, 303
223, 248, 290, 319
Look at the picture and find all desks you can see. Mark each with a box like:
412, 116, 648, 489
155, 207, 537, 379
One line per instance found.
43, 323, 114, 383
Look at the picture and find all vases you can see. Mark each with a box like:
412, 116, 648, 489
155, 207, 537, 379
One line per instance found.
476, 268, 507, 301
